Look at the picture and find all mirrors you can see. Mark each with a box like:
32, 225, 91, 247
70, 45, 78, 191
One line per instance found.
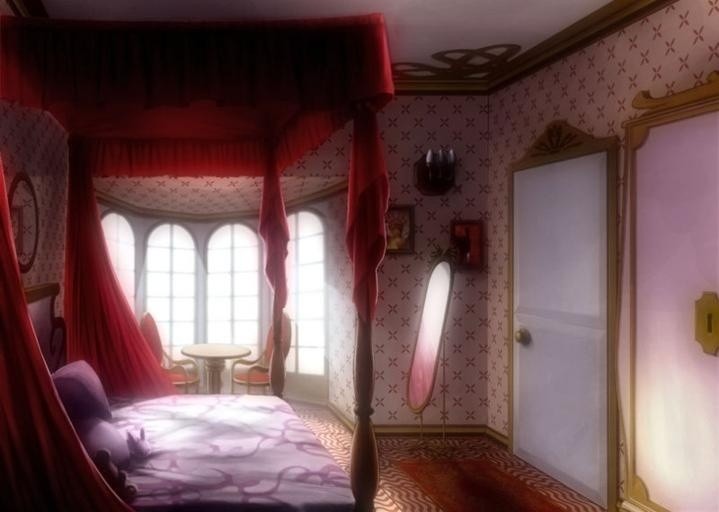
405, 255, 455, 450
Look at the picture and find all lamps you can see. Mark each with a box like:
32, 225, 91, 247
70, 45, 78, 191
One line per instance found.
413, 148, 456, 196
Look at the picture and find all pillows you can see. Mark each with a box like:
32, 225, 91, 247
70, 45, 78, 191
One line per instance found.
49, 359, 150, 466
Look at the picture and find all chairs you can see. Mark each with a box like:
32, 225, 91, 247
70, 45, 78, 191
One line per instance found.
139, 313, 200, 395
230, 313, 292, 394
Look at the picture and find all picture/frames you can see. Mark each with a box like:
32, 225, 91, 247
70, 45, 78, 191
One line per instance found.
450, 219, 484, 270
384, 204, 415, 254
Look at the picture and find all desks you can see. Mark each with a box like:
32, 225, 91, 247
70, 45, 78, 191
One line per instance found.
182, 344, 252, 393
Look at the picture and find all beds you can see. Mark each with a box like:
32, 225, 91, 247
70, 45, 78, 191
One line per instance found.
0, 281, 355, 512
